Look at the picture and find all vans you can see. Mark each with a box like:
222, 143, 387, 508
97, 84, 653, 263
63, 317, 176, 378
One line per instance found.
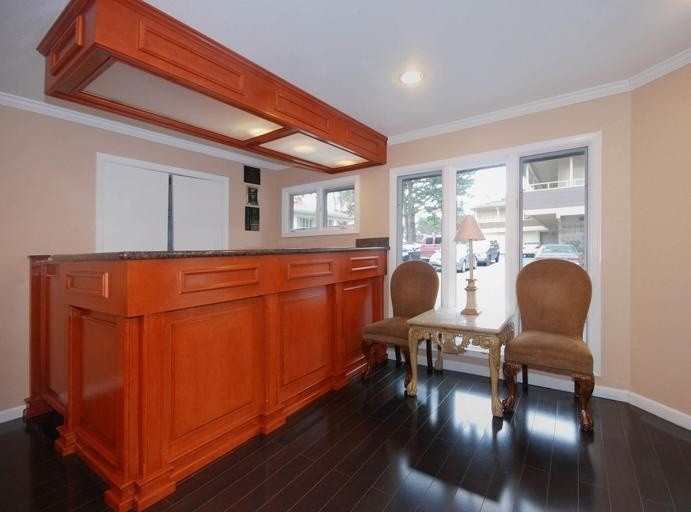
421, 235, 442, 261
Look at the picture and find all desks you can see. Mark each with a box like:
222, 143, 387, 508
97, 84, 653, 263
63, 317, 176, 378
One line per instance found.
406, 309, 515, 418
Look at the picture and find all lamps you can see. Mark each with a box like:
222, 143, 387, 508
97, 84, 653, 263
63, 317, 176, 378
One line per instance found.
452, 215, 485, 316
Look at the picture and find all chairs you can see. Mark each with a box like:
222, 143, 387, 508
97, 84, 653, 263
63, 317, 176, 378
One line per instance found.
501, 256, 595, 433
359, 260, 440, 388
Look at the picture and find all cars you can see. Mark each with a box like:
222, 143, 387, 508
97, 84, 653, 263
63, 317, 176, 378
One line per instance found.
535, 244, 584, 269
429, 244, 478, 272
402, 238, 421, 259
522, 241, 540, 256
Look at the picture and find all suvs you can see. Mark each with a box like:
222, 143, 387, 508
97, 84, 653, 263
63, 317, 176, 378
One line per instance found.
473, 240, 500, 266
